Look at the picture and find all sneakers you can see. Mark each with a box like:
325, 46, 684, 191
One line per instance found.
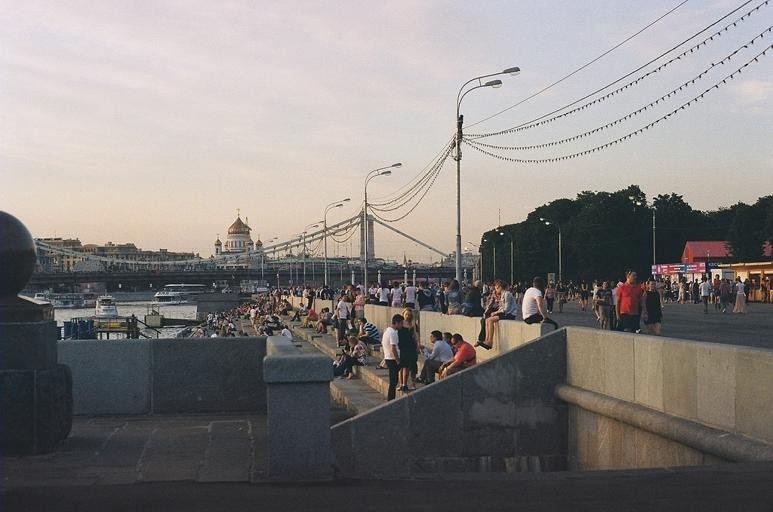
547, 309, 552, 313
397, 383, 417, 390
474, 340, 492, 349
415, 377, 435, 385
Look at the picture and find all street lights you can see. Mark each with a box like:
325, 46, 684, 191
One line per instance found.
261, 237, 278, 279
302, 220, 324, 288
628, 194, 657, 265
454, 66, 520, 286
364, 162, 403, 302
539, 217, 562, 282
324, 197, 351, 286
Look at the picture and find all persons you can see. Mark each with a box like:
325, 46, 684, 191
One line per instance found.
416, 330, 476, 385
377, 307, 420, 402
192, 274, 560, 350
343, 337, 366, 379
561, 267, 772, 336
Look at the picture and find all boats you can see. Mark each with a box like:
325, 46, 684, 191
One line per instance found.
95, 289, 118, 318
33, 291, 96, 308
151, 283, 207, 304
